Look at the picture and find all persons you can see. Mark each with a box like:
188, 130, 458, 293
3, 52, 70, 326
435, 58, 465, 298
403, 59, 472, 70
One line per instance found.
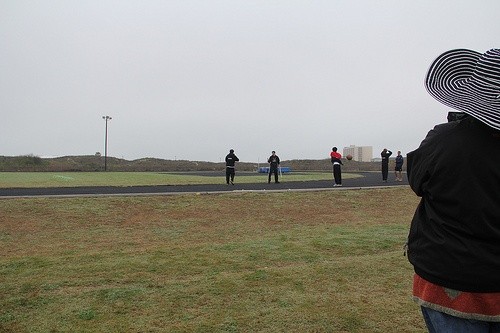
267, 151, 281, 184
330, 147, 344, 187
394, 151, 404, 181
380, 150, 392, 182
224, 149, 239, 186
406, 48, 500, 333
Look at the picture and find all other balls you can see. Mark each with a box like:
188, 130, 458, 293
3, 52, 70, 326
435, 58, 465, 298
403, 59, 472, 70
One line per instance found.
346, 154, 353, 161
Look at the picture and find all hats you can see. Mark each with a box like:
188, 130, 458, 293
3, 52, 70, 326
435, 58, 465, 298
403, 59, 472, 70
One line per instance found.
426, 48, 500, 130
